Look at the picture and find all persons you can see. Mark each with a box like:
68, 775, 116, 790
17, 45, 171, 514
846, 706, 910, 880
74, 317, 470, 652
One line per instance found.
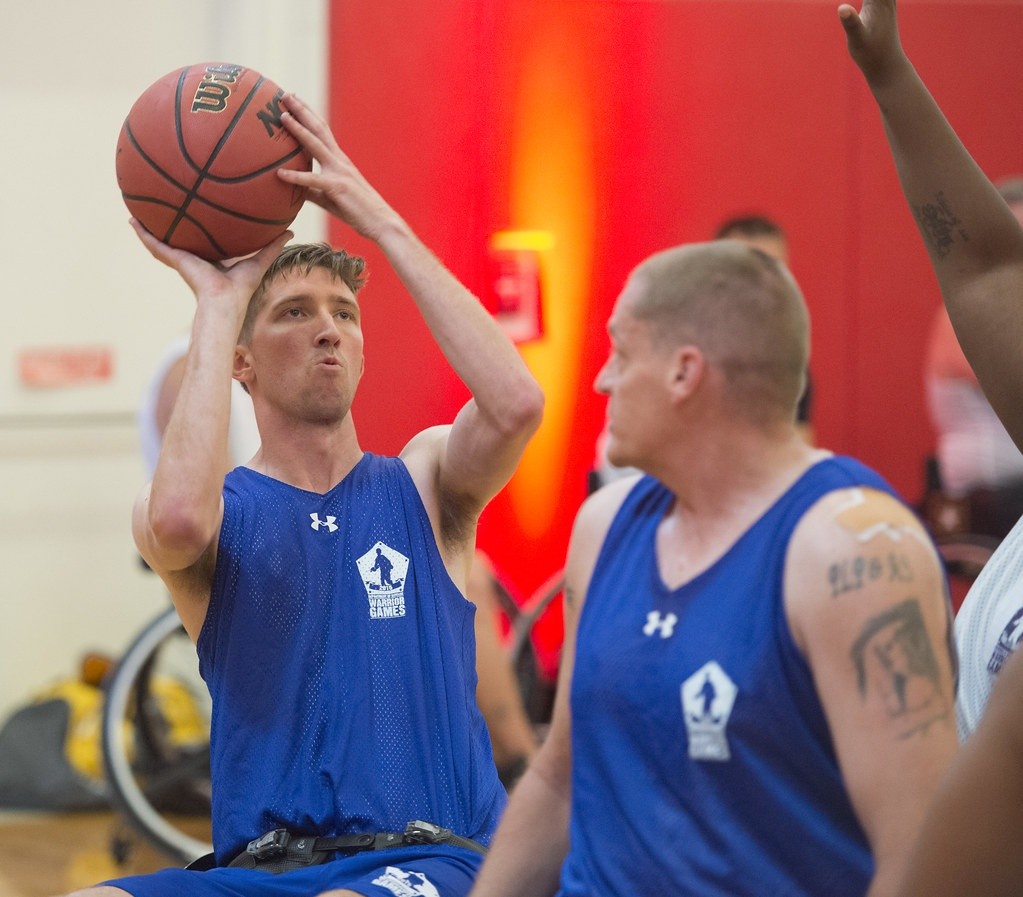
66, 88, 548, 896
459, 0, 1023, 897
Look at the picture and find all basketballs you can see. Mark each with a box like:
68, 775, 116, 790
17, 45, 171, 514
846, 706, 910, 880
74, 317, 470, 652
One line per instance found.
114, 61, 314, 262
